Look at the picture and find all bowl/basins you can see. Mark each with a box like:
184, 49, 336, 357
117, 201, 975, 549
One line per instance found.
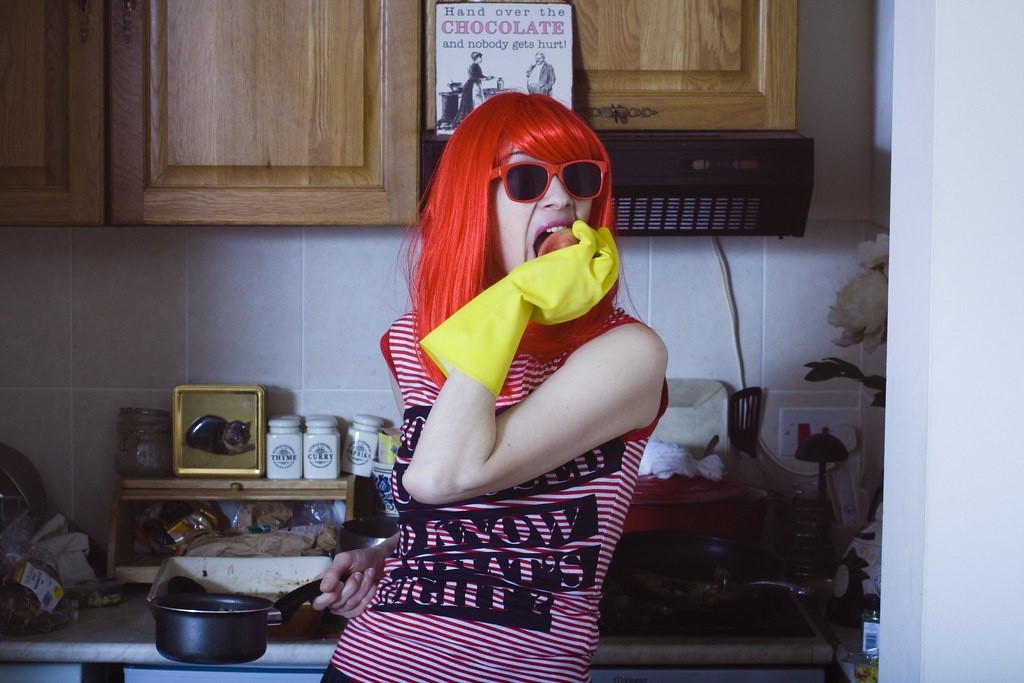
371, 459, 400, 517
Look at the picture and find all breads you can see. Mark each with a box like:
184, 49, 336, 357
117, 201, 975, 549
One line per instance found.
0, 559, 75, 637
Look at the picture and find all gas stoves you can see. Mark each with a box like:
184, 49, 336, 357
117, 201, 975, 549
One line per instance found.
305, 574, 834, 683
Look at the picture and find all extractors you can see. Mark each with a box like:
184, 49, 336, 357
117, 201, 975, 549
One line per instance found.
420, 128, 814, 238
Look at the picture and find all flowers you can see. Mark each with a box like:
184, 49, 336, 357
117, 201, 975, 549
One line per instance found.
803, 231, 887, 411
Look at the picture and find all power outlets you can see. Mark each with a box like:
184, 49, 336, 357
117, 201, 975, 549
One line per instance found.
778, 407, 861, 456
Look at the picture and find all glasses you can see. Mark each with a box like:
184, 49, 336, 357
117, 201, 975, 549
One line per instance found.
490, 159, 608, 203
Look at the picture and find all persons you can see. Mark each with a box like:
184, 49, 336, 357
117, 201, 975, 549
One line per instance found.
308, 91, 669, 683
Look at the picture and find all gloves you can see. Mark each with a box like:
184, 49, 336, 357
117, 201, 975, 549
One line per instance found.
420, 221, 622, 399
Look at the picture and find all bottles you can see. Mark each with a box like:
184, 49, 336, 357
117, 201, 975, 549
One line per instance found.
267, 415, 302, 479
303, 417, 340, 480
112, 406, 175, 480
859, 593, 880, 660
340, 414, 384, 478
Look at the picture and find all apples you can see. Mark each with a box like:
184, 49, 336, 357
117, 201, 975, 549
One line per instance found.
537, 227, 580, 257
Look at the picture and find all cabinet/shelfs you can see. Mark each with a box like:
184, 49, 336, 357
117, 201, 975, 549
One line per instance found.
0, 0, 798, 228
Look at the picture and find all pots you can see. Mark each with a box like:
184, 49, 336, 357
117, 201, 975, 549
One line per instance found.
605, 529, 814, 607
146, 576, 348, 665
